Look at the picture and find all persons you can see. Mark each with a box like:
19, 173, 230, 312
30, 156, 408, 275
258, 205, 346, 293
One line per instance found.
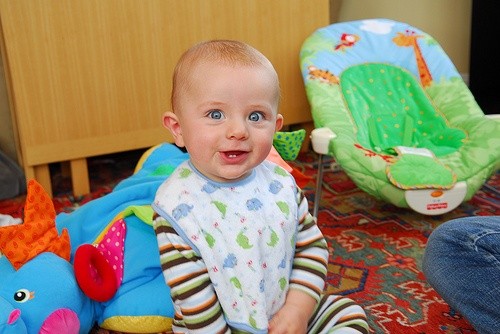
423, 216, 499, 334
153, 39, 371, 334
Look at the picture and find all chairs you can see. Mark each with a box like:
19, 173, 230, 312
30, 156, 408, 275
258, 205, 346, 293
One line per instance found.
298, 17, 500, 227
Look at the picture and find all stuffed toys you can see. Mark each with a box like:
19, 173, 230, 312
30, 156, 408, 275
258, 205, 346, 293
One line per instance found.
0, 140, 181, 333
